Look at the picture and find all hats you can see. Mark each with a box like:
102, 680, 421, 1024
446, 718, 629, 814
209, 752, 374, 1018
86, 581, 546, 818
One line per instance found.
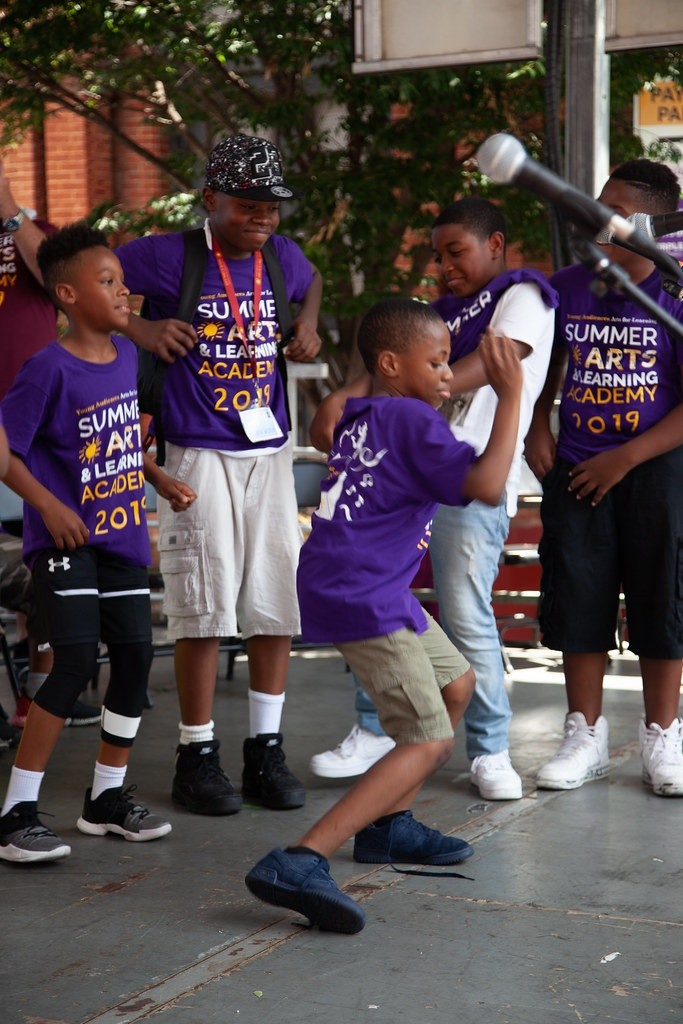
204, 135, 296, 201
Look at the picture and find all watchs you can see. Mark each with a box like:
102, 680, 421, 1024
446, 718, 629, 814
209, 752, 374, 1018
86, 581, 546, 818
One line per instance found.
2, 209, 23, 231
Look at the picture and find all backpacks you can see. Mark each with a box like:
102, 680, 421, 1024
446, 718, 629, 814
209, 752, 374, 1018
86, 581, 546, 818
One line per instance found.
133, 229, 293, 465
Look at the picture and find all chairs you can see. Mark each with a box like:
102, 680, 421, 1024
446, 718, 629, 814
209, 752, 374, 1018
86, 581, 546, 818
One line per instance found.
0, 449, 331, 756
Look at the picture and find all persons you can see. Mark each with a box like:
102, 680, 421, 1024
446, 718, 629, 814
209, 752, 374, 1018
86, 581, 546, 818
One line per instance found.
245, 299, 522, 931
0, 220, 197, 862
0, 161, 104, 748
523, 159, 682, 800
308, 201, 557, 802
111, 133, 319, 816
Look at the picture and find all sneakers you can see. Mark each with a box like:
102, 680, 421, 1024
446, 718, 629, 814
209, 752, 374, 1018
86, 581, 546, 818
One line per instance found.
353, 811, 476, 881
0, 802, 72, 863
245, 848, 365, 934
640, 713, 683, 795
309, 725, 396, 778
171, 740, 242, 814
76, 786, 172, 841
12, 687, 102, 726
535, 711, 610, 790
0, 707, 16, 747
470, 750, 522, 800
243, 734, 305, 807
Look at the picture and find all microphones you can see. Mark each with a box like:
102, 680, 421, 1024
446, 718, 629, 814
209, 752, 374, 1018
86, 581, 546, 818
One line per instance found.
476, 132, 683, 279
